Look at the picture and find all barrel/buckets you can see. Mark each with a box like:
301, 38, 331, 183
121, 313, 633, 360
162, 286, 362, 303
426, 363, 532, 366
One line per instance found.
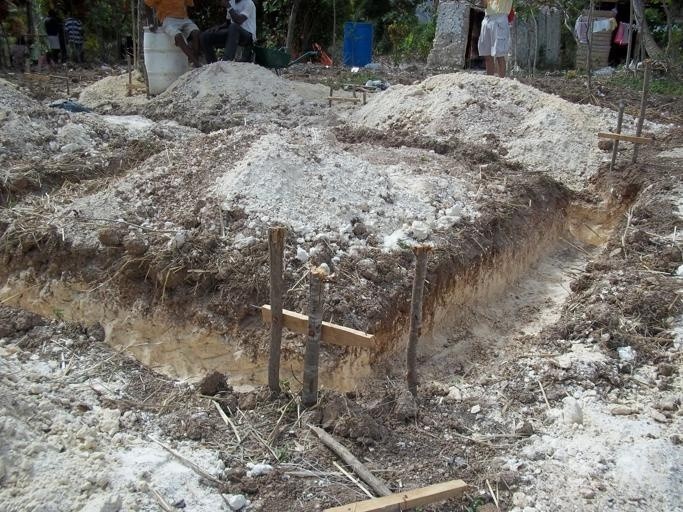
142, 26, 188, 96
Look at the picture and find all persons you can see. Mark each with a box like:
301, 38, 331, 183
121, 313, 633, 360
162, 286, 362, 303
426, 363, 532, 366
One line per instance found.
45, 10, 65, 65
27, 39, 38, 65
64, 12, 84, 63
477, 0, 513, 77
200, 1, 257, 63
10, 33, 29, 74
144, 0, 202, 68
